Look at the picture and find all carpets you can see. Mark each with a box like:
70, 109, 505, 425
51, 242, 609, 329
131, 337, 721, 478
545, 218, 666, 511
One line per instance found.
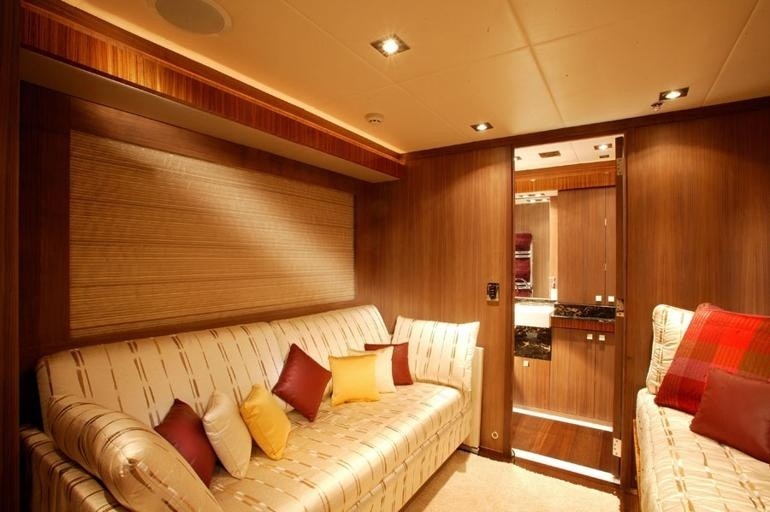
399, 450, 624, 512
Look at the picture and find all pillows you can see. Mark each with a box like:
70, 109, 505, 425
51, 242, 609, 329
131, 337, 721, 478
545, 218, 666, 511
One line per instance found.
325, 350, 385, 405
690, 365, 770, 464
644, 303, 695, 395
153, 396, 221, 488
199, 388, 254, 480
272, 343, 334, 423
347, 346, 395, 398
393, 313, 481, 392
239, 382, 291, 461
654, 301, 770, 415
364, 342, 415, 388
42, 391, 227, 512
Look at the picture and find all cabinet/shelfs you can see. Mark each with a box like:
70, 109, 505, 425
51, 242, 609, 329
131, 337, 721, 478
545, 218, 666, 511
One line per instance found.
551, 327, 616, 422
557, 186, 617, 306
513, 355, 550, 416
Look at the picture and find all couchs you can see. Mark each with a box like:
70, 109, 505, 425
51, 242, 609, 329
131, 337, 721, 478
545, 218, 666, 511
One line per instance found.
631, 300, 770, 512
19, 304, 486, 512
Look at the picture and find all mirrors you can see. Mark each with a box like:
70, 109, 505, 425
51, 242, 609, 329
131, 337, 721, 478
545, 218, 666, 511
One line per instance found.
513, 192, 559, 302
